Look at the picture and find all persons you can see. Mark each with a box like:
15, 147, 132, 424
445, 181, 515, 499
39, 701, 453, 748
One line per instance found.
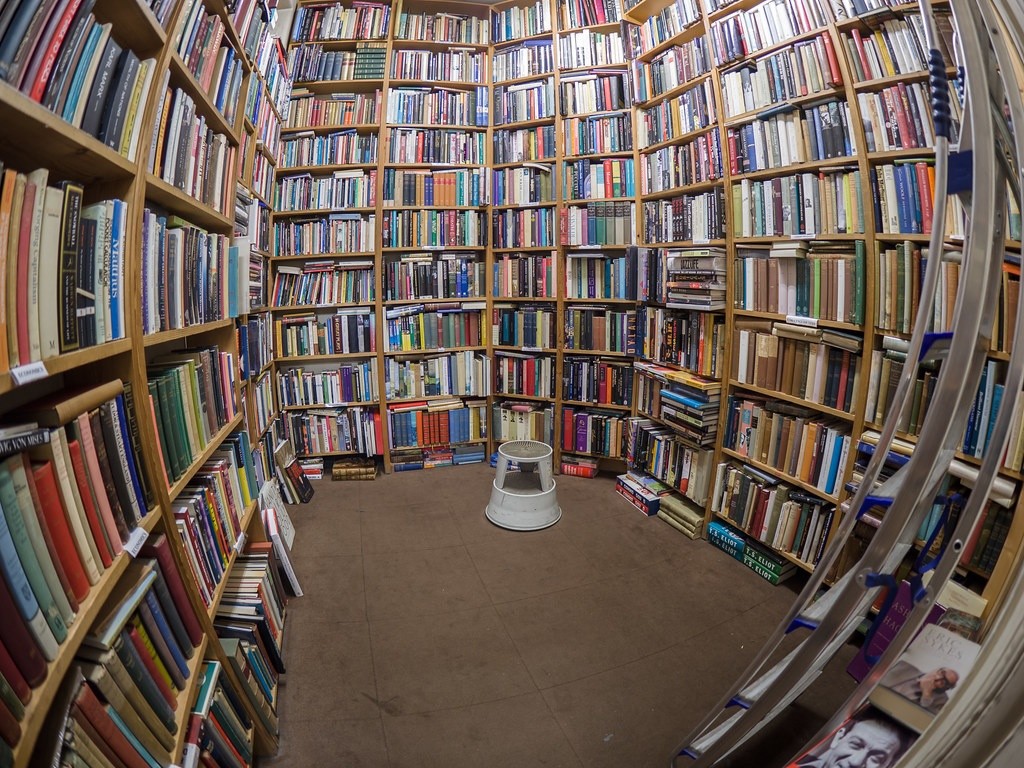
886, 658, 960, 716
801, 702, 916, 768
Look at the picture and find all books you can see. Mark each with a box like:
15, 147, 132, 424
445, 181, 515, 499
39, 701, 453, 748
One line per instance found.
2, 1, 1024, 767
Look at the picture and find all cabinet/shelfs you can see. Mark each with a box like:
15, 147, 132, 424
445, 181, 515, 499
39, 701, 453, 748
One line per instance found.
0, 0, 1024, 768
